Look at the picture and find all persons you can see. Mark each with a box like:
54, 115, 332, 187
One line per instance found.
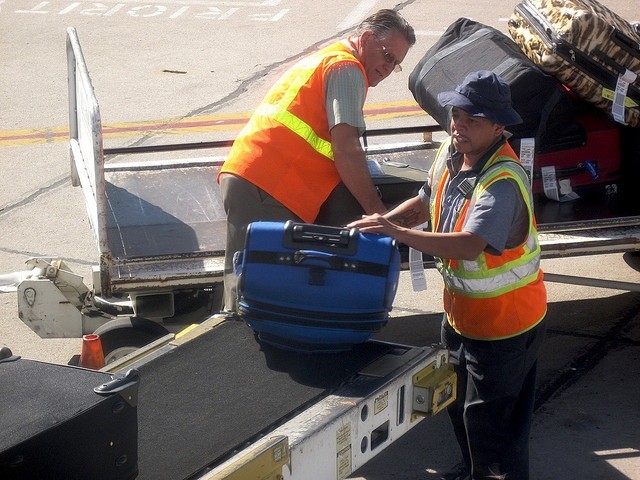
341, 69, 549, 478
216, 7, 416, 314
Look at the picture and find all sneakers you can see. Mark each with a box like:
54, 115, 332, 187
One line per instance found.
439, 458, 471, 480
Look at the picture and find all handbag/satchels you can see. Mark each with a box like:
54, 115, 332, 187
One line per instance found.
407, 17, 586, 157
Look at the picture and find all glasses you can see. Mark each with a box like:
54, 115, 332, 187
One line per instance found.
371, 31, 405, 74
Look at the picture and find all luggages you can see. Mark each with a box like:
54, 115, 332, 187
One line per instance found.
507, 0, 640, 133
549, 157, 640, 219
233, 219, 402, 359
520, 117, 620, 200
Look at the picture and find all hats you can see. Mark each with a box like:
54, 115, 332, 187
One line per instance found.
436, 69, 524, 129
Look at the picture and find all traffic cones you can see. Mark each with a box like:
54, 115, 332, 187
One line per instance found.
78, 334, 106, 370
